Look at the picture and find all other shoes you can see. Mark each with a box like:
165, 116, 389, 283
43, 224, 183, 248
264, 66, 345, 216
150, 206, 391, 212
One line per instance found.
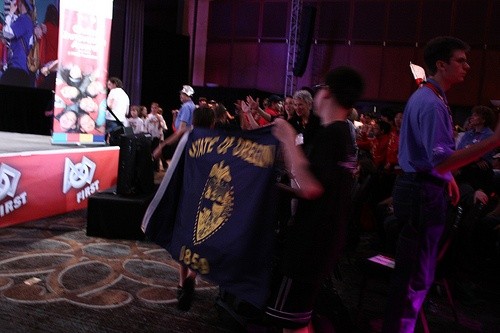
186, 276, 195, 292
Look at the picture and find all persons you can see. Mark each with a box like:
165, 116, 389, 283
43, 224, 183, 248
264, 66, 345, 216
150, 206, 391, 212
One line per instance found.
0, 0, 59, 88
106, 78, 500, 306
381, 35, 500, 332
265, 66, 367, 333
53, 65, 107, 135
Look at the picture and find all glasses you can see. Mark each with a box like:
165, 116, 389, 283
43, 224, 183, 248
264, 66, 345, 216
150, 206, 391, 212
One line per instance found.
312, 84, 329, 92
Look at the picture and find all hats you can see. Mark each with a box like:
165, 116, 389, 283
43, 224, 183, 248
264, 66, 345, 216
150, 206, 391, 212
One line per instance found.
182, 84, 195, 96
